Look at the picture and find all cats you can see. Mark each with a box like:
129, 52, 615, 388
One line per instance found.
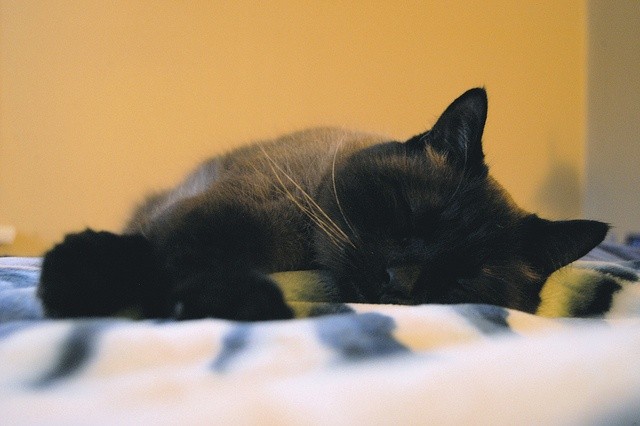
36, 85, 611, 320
